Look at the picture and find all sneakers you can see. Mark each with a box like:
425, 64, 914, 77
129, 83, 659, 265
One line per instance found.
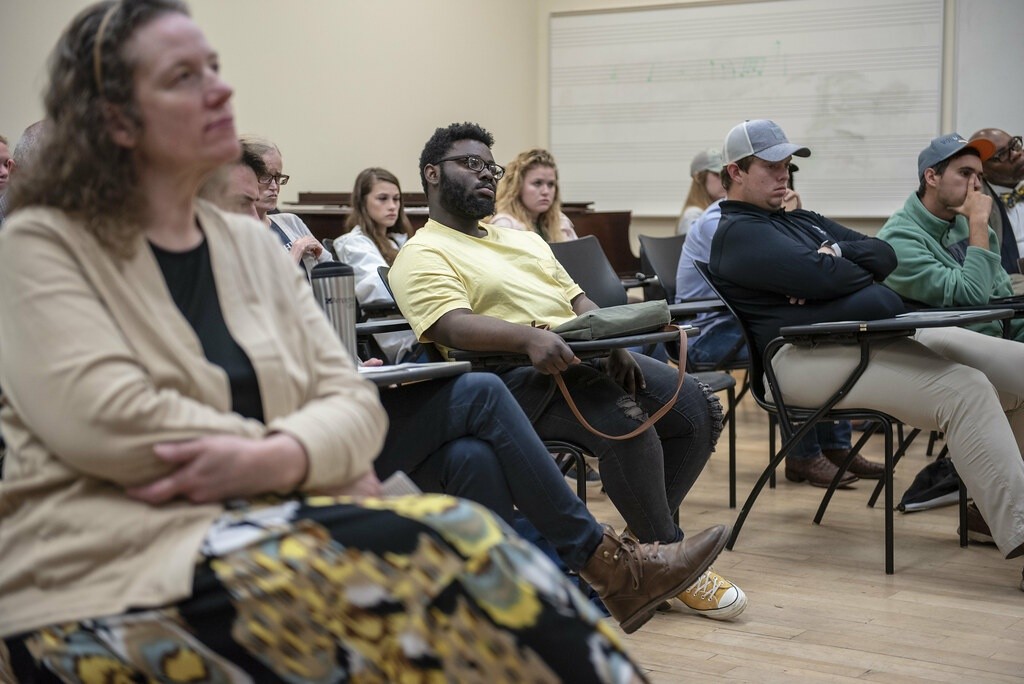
556, 458, 603, 487
676, 570, 748, 617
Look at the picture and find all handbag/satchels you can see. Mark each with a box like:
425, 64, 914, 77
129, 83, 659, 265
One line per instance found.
549, 298, 672, 339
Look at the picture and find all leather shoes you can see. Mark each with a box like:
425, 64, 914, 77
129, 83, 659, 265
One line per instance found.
822, 447, 895, 478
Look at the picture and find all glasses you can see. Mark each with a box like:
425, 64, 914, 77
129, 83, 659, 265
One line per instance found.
258, 173, 289, 185
432, 155, 506, 180
988, 135, 1023, 163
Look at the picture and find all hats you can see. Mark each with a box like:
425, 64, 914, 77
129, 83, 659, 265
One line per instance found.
690, 150, 724, 177
918, 132, 997, 182
723, 119, 811, 167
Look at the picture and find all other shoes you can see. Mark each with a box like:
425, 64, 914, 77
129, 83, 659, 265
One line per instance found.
785, 453, 859, 486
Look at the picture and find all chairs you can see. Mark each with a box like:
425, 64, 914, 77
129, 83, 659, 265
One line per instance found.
299, 198, 1024, 579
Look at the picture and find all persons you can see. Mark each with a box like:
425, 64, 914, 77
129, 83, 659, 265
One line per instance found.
225, 142, 730, 635
0, 120, 46, 223
707, 119, 1024, 593
388, 122, 748, 619
333, 167, 419, 366
969, 127, 1024, 275
481, 148, 577, 242
675, 187, 894, 487
0, 0, 647, 684
873, 132, 1024, 343
677, 150, 727, 236
247, 139, 332, 274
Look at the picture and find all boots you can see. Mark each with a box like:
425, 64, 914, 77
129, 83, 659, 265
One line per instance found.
578, 522, 733, 635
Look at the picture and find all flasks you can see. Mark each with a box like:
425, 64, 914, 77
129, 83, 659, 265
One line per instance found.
311, 262, 357, 370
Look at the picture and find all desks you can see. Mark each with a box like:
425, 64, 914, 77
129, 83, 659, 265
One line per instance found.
561, 315, 700, 345
352, 319, 420, 335
783, 306, 1016, 351
668, 298, 729, 318
355, 356, 473, 387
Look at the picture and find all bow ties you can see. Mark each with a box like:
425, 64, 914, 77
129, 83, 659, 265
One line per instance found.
999, 185, 1024, 209
